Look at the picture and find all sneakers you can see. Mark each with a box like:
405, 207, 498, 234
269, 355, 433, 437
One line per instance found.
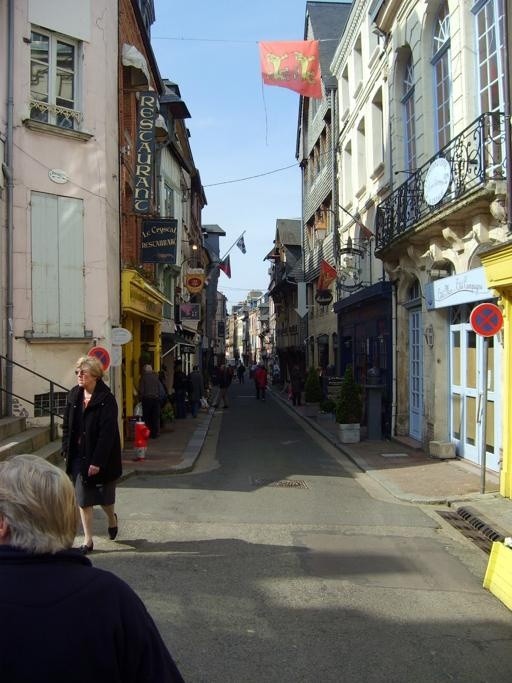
256, 397, 266, 401
211, 404, 229, 409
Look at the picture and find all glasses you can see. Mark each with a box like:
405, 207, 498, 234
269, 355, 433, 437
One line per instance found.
74, 370, 93, 376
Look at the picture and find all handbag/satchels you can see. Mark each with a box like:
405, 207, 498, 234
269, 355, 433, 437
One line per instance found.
199, 397, 208, 409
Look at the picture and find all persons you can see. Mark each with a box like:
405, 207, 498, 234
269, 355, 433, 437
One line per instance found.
290, 365, 304, 406
188, 367, 204, 418
212, 363, 267, 409
173, 365, 188, 418
139, 365, 160, 438
316, 364, 326, 384
60, 356, 122, 554
0, 455, 186, 683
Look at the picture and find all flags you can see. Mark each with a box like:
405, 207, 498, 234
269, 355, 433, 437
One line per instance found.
237, 237, 247, 254
219, 255, 231, 278
258, 40, 322, 100
318, 261, 336, 290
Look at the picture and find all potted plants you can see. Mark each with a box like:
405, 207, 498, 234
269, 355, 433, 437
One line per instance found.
160, 406, 176, 431
302, 365, 322, 417
334, 363, 363, 444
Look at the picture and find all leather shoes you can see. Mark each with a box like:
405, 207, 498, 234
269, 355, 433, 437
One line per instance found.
78, 542, 94, 555
108, 513, 119, 540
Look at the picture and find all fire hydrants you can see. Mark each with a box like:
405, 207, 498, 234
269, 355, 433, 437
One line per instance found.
132, 416, 151, 462
